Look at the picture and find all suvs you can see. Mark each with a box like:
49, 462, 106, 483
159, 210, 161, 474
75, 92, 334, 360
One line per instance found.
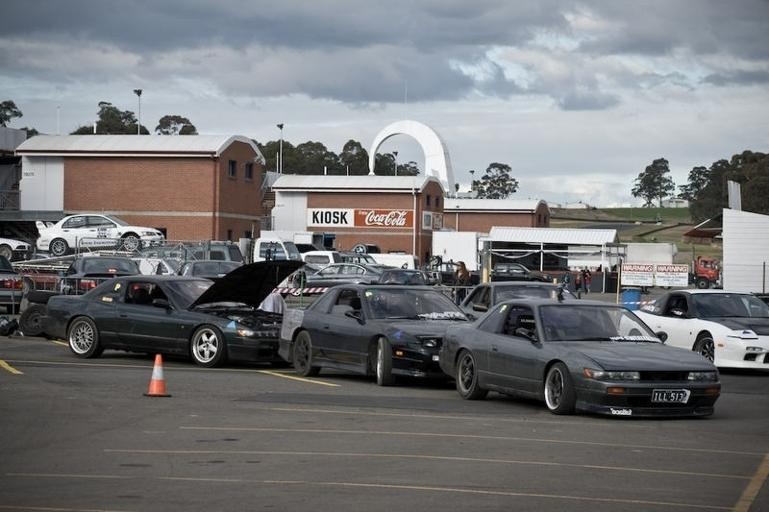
490, 263, 552, 281
306, 263, 400, 284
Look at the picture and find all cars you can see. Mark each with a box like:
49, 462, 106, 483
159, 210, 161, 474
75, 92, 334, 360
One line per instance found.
439, 299, 721, 417
436, 262, 479, 285
0, 238, 32, 263
288, 285, 473, 385
458, 282, 582, 322
0, 256, 26, 314
43, 260, 307, 367
63, 257, 142, 296
36, 213, 165, 256
377, 270, 432, 284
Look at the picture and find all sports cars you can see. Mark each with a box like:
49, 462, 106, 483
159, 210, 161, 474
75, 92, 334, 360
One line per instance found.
619, 290, 769, 368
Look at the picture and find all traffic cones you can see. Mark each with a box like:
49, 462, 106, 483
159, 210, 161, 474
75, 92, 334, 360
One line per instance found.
143, 354, 172, 397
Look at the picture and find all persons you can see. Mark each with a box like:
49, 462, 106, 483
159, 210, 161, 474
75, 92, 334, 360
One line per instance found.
575, 271, 582, 292
452, 261, 469, 304
583, 265, 590, 290
585, 272, 592, 294
564, 271, 571, 290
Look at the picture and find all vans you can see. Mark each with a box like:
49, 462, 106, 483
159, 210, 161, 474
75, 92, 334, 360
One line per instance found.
127, 256, 191, 278
342, 255, 377, 264
302, 249, 342, 271
302, 251, 342, 263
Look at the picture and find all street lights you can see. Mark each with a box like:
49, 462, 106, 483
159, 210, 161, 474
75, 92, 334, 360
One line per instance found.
134, 89, 142, 134
394, 151, 398, 175
470, 170, 474, 190
277, 124, 283, 173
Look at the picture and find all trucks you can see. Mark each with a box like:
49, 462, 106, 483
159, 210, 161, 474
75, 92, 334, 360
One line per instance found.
688, 256, 720, 289
171, 238, 302, 290
